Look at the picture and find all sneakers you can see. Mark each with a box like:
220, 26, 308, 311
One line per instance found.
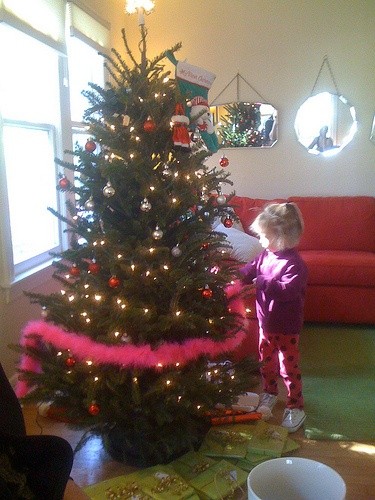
257, 392, 278, 415
279, 408, 306, 433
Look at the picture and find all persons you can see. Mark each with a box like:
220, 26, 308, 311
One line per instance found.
222, 202, 306, 433
0, 359, 74, 500
307, 126, 340, 154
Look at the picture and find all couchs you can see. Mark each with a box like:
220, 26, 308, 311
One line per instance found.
212, 194, 375, 322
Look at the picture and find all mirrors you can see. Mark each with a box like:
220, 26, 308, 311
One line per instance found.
294, 54, 359, 156
208, 73, 279, 148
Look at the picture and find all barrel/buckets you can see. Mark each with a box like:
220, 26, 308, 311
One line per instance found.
247, 457, 346, 500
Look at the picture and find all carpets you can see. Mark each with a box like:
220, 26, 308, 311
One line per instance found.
299, 324, 375, 441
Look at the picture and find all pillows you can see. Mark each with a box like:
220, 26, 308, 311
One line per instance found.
212, 207, 265, 263
